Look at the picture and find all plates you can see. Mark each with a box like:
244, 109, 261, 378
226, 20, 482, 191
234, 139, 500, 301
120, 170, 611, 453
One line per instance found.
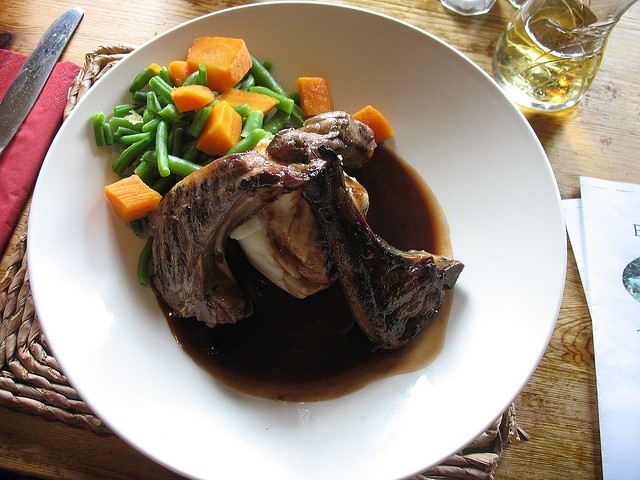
26, 0, 568, 480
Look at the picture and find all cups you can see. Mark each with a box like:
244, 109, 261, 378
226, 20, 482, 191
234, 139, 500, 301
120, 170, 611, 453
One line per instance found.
491, 1, 636, 114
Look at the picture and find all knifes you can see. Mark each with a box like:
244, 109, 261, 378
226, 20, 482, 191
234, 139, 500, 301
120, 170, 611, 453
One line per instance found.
1, 6, 86, 152
